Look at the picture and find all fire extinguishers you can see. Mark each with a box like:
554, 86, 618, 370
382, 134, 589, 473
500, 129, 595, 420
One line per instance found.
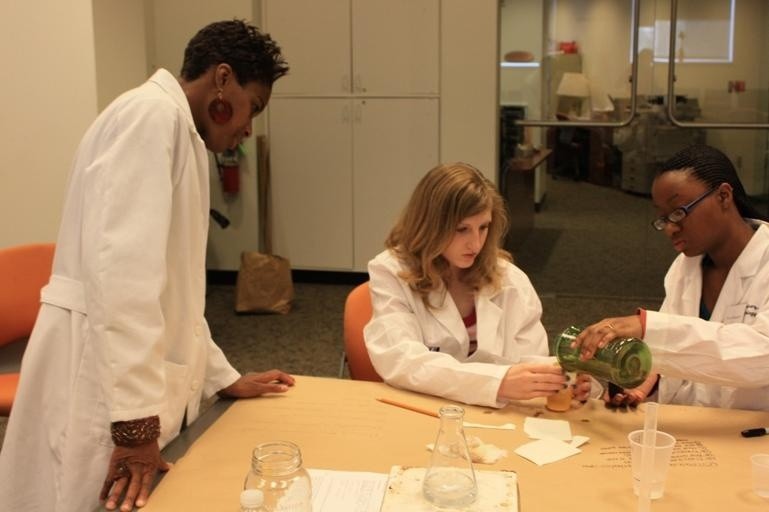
213, 148, 240, 193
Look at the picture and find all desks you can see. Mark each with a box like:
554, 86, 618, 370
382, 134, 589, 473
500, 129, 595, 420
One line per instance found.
503, 147, 554, 251
92, 374, 768, 511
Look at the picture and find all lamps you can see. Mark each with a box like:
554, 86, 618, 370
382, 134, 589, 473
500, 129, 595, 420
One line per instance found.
555, 72, 590, 122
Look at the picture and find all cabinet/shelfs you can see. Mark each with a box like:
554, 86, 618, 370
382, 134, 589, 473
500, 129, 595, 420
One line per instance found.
256, 1, 439, 97
619, 119, 706, 200
439, 2, 503, 266
260, 96, 441, 287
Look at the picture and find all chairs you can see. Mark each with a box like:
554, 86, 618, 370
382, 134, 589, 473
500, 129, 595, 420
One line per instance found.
345, 283, 387, 382
0, 242, 57, 418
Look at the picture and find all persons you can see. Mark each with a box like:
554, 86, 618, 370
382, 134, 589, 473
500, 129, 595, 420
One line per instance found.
2, 21, 296, 512
571, 147, 767, 414
360, 160, 593, 408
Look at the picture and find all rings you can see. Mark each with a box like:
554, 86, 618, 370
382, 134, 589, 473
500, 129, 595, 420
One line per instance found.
114, 465, 128, 480
605, 324, 615, 330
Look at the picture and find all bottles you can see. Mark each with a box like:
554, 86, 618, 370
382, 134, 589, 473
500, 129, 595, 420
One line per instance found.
515, 142, 543, 163
244, 440, 315, 512
237, 489, 269, 512
425, 406, 478, 507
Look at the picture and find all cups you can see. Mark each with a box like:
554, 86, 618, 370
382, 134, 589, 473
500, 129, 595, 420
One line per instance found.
561, 43, 575, 53
752, 451, 768, 496
544, 372, 575, 410
557, 323, 652, 389
729, 80, 745, 93
628, 430, 679, 503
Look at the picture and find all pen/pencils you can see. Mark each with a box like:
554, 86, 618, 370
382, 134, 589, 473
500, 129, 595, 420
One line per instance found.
741, 427, 769, 436
375, 397, 441, 418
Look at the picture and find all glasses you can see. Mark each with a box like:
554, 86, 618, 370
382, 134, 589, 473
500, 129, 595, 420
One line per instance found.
652, 186, 722, 232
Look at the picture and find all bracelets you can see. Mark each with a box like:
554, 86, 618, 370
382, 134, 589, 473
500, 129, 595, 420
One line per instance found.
108, 417, 161, 447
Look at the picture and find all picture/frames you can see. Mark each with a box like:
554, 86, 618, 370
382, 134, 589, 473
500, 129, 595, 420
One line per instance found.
653, 0, 735, 62
